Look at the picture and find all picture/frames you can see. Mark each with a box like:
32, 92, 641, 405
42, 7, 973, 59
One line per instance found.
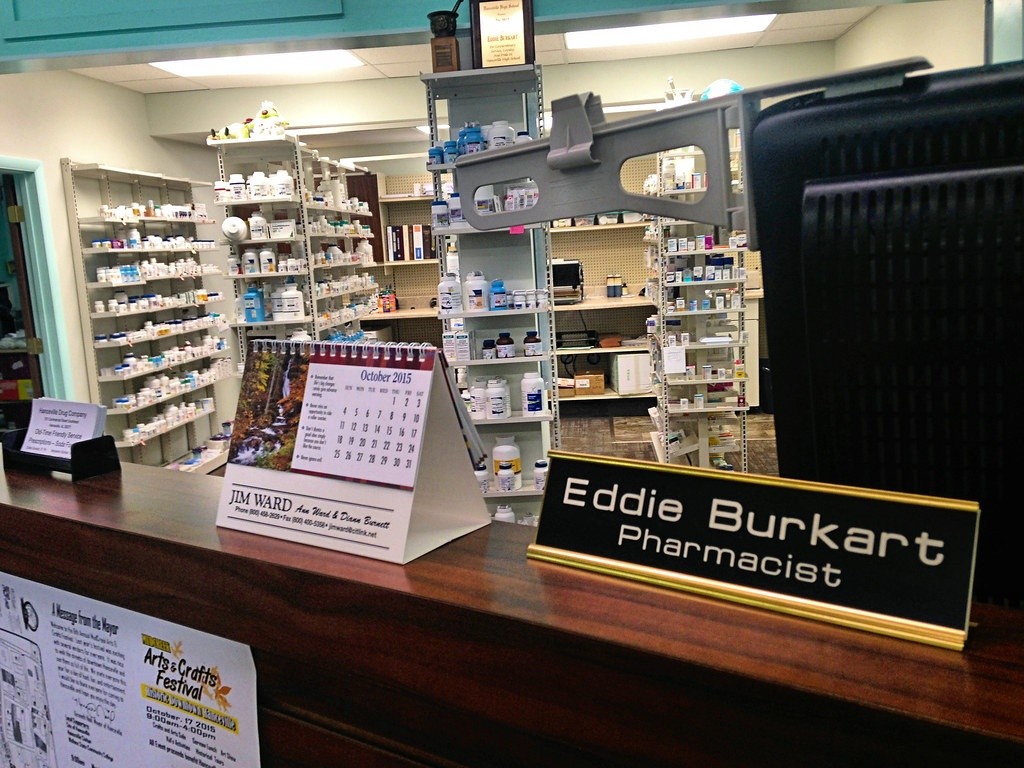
469, 0, 536, 69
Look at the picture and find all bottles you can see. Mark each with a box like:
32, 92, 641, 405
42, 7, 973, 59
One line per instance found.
521, 373, 546, 417
498, 463, 514, 492
495, 504, 515, 523
482, 340, 496, 359
535, 459, 548, 491
490, 279, 506, 311
474, 464, 489, 493
524, 331, 542, 356
428, 121, 532, 165
493, 434, 522, 491
438, 250, 462, 314
622, 282, 628, 296
496, 333, 515, 358
507, 290, 546, 310
457, 369, 511, 420
92, 170, 396, 442
520, 513, 535, 526
465, 276, 489, 311
431, 193, 538, 228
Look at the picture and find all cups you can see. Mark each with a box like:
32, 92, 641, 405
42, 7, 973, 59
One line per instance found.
427, 10, 459, 37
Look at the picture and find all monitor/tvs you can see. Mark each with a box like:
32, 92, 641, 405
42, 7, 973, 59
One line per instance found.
754, 61, 1024, 608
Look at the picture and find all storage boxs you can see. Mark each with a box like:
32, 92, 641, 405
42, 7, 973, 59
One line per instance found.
598, 212, 618, 224
574, 370, 605, 395
623, 210, 645, 223
574, 214, 595, 226
608, 353, 653, 396
0, 380, 34, 400
553, 218, 572, 227
557, 377, 575, 398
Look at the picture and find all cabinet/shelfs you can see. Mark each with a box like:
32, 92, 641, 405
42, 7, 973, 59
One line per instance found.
207, 132, 378, 380
642, 114, 753, 475
0, 282, 35, 405
60, 156, 232, 476
726, 299, 761, 407
418, 64, 563, 528
340, 97, 656, 410
